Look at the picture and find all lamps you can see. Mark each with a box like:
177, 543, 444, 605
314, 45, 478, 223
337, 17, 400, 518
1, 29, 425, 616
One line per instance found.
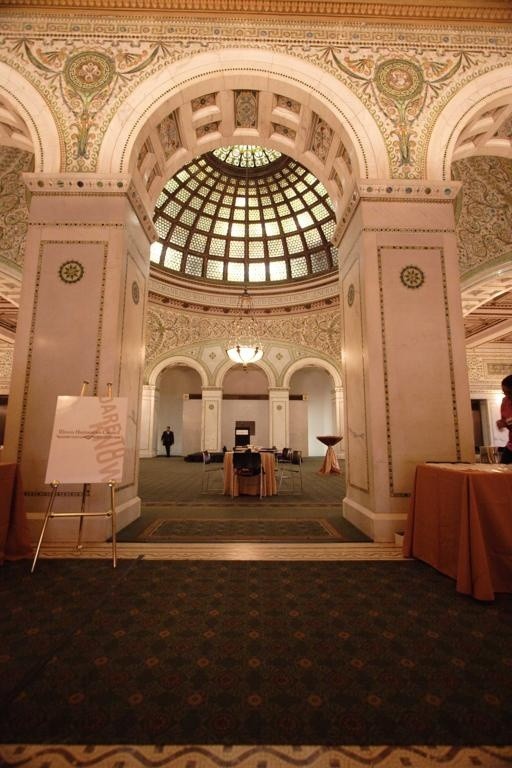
225, 287, 264, 372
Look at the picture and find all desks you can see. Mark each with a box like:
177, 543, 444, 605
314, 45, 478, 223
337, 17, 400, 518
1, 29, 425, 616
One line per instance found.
415, 461, 511, 601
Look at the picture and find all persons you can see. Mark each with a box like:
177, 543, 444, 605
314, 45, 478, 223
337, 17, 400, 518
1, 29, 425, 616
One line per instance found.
161, 425, 174, 456
496, 372, 512, 464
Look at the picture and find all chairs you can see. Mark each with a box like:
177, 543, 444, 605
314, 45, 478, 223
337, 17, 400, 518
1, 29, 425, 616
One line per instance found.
201, 443, 304, 499
